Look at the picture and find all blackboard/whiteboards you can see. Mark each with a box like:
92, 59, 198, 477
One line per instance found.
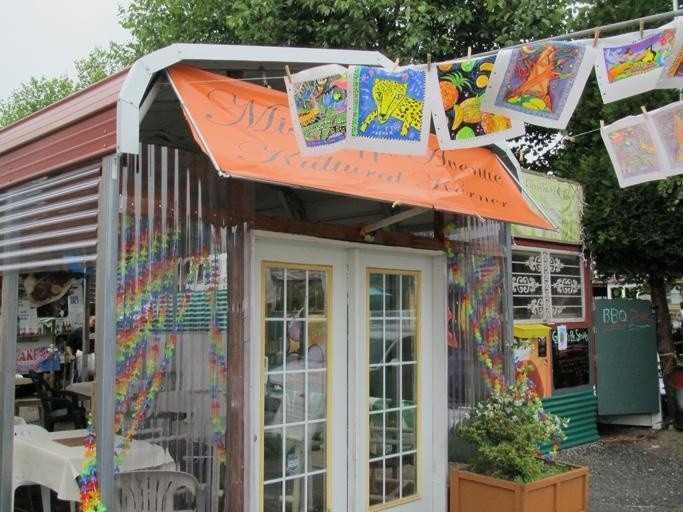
551, 328, 589, 388
596, 300, 659, 415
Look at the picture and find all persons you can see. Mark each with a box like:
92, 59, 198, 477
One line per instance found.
65, 314, 96, 376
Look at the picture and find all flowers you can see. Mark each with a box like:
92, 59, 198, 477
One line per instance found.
454, 379, 570, 479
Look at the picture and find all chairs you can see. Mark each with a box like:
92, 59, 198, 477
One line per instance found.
11, 424, 76, 512
28, 369, 87, 432
114, 470, 208, 512
130, 411, 187, 440
15, 369, 54, 427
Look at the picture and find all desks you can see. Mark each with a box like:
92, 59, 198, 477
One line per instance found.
63, 382, 95, 414
13, 429, 175, 512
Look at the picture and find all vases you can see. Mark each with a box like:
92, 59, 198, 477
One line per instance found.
450, 457, 589, 512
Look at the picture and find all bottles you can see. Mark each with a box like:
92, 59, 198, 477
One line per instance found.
557, 324, 568, 351
